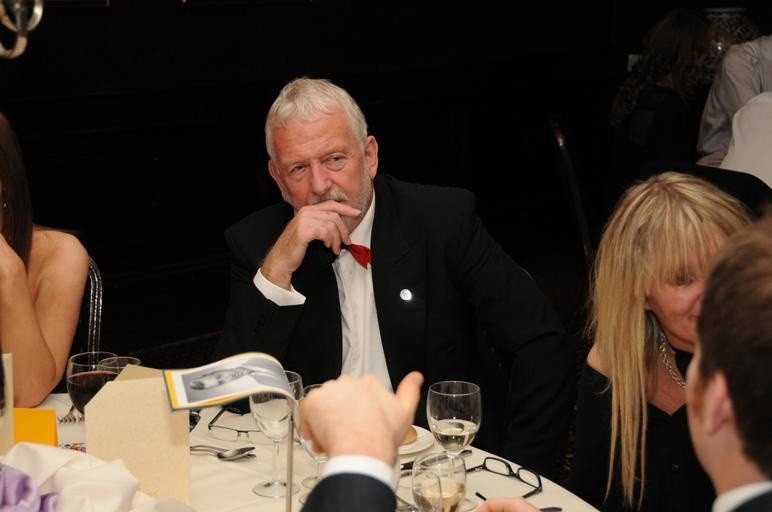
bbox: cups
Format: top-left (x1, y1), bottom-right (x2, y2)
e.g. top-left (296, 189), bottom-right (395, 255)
top-left (66, 351), bottom-right (142, 415)
top-left (411, 452), bottom-right (467, 512)
top-left (388, 470), bottom-right (442, 512)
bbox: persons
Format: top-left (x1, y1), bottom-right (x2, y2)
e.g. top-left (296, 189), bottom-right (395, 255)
top-left (0, 113), bottom-right (90, 409)
top-left (550, 168), bottom-right (755, 512)
top-left (290, 212), bottom-right (771, 510)
top-left (609, 8), bottom-right (772, 191)
top-left (187, 354), bottom-right (292, 392)
top-left (214, 75), bottom-right (580, 481)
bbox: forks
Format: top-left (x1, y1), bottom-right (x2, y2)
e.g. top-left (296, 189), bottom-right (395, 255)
top-left (57, 404), bottom-right (77, 424)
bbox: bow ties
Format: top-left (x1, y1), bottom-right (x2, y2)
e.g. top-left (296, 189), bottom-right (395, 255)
top-left (327, 242), bottom-right (371, 269)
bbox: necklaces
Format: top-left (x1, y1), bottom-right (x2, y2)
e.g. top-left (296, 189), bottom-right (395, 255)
top-left (657, 332), bottom-right (687, 392)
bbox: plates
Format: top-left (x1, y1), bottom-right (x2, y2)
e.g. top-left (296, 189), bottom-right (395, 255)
top-left (398, 425), bottom-right (434, 454)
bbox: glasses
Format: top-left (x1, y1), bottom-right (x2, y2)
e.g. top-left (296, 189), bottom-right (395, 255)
top-left (207, 404), bottom-right (290, 447)
top-left (427, 458), bottom-right (543, 503)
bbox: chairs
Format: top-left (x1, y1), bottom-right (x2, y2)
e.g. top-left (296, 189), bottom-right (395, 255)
top-left (68, 258), bottom-right (107, 365)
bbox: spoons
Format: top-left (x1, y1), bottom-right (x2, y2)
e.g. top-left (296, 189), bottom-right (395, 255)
top-left (190, 446), bottom-right (256, 461)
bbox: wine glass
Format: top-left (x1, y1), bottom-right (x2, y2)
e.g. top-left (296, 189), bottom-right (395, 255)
top-left (249, 370), bottom-right (304, 498)
top-left (426, 381), bottom-right (482, 512)
top-left (299, 384), bottom-right (330, 490)
